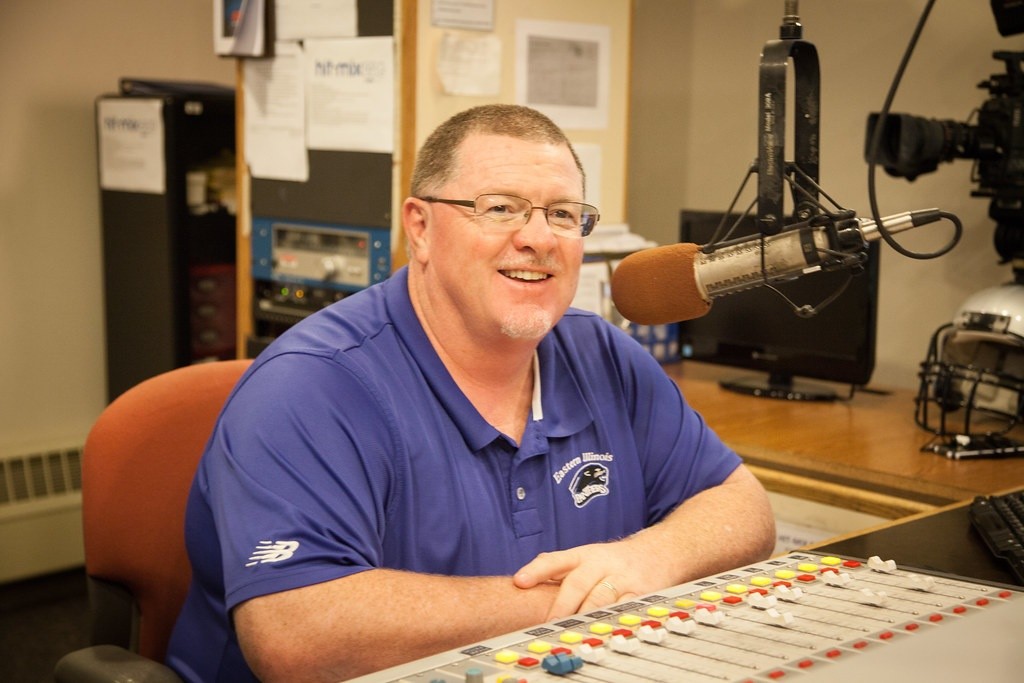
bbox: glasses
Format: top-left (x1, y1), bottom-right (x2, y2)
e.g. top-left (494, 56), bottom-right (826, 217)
top-left (417, 192), bottom-right (601, 239)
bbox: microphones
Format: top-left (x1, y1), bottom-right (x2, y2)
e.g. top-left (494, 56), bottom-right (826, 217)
top-left (610, 206), bottom-right (941, 326)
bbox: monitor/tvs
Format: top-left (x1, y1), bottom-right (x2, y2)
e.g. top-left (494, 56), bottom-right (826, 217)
top-left (677, 206), bottom-right (880, 401)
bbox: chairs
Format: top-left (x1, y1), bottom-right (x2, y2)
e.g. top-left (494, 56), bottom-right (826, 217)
top-left (80, 359), bottom-right (254, 667)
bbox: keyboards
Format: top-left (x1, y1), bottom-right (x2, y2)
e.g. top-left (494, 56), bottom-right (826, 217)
top-left (968, 488), bottom-right (1024, 588)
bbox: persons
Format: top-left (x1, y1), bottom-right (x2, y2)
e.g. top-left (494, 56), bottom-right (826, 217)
top-left (169, 105), bottom-right (778, 683)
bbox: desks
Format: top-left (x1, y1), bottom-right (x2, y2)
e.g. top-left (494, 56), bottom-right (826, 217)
top-left (648, 352), bottom-right (1024, 559)
